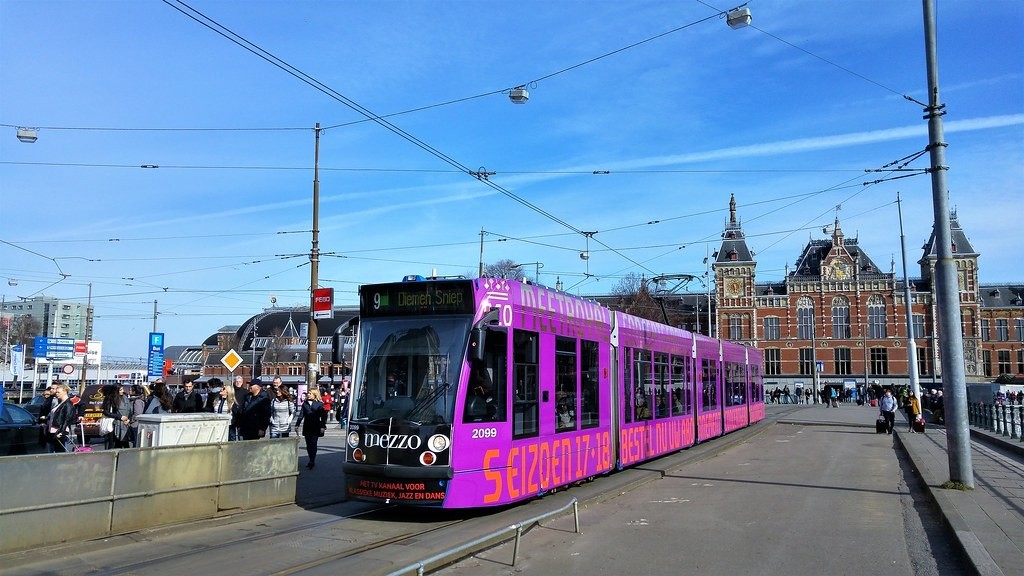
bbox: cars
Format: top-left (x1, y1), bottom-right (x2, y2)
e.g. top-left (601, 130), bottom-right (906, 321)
top-left (0, 401), bottom-right (49, 457)
top-left (23, 394), bottom-right (48, 418)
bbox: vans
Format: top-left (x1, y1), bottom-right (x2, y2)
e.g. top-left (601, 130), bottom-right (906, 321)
top-left (73, 384), bottom-right (152, 445)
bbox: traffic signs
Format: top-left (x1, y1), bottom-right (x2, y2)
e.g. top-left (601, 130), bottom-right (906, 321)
top-left (313, 288), bottom-right (335, 320)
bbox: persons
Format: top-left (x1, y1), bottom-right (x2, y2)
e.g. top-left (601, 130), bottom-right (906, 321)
top-left (23, 375), bottom-right (355, 471)
top-left (765, 381), bottom-right (945, 423)
top-left (879, 387), bottom-right (898, 435)
top-left (904, 389), bottom-right (921, 433)
top-left (993, 389), bottom-right (1024, 405)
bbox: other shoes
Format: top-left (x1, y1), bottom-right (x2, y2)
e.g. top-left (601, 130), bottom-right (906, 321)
top-left (306, 462), bottom-right (314, 469)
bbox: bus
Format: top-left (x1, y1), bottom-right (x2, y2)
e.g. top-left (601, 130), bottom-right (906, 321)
top-left (332, 268), bottom-right (765, 507)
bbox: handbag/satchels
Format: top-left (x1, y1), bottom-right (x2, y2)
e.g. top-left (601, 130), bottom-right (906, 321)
top-left (99, 406), bottom-right (113, 436)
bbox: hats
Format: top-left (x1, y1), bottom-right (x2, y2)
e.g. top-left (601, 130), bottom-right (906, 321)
top-left (246, 378), bottom-right (263, 387)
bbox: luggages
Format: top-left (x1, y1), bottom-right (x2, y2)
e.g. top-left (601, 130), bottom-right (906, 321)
top-left (871, 396), bottom-right (876, 406)
top-left (833, 399), bottom-right (838, 407)
top-left (913, 413), bottom-right (925, 433)
top-left (876, 415), bottom-right (886, 434)
top-left (55, 434), bottom-right (93, 453)
